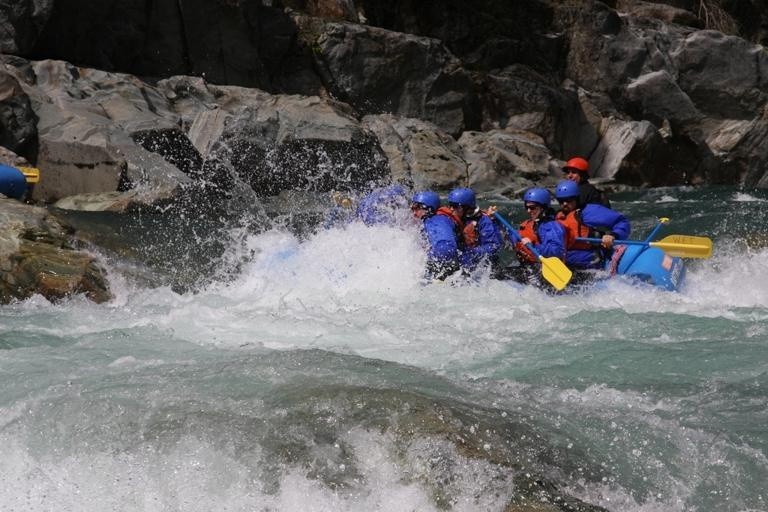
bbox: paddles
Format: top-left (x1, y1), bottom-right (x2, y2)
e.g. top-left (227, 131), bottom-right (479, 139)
top-left (16, 167), bottom-right (40, 184)
top-left (491, 213), bottom-right (572, 291)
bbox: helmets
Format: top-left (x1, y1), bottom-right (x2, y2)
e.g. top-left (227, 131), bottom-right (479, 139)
top-left (448, 187), bottom-right (475, 209)
top-left (524, 186), bottom-right (551, 206)
top-left (555, 179), bottom-right (580, 199)
top-left (412, 191), bottom-right (440, 211)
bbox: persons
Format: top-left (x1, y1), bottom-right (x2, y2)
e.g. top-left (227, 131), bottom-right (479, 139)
top-left (562, 156), bottom-right (611, 212)
top-left (325, 181), bottom-right (505, 285)
top-left (552, 179), bottom-right (631, 277)
top-left (485, 187), bottom-right (566, 298)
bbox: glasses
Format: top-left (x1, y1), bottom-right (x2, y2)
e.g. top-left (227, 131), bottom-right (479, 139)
top-left (450, 203), bottom-right (463, 208)
top-left (563, 157), bottom-right (589, 172)
top-left (525, 204), bottom-right (540, 211)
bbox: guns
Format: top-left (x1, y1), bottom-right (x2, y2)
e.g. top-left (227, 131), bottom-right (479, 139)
top-left (576, 235), bottom-right (713, 260)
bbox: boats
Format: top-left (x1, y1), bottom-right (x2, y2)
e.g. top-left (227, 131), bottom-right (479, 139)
top-left (0, 165), bottom-right (27, 199)
top-left (255, 238), bottom-right (683, 296)
